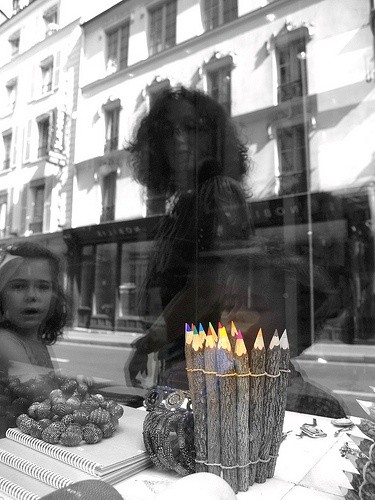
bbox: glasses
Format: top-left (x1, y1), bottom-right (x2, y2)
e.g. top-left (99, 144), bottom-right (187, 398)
top-left (158, 119), bottom-right (205, 139)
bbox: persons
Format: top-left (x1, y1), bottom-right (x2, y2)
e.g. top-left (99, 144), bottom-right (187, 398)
top-left (0, 242), bottom-right (75, 373)
top-left (126, 88), bottom-right (251, 396)
top-left (203, 239), bottom-right (343, 420)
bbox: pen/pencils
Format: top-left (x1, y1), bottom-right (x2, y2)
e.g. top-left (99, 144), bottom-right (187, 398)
top-left (180, 320), bottom-right (293, 493)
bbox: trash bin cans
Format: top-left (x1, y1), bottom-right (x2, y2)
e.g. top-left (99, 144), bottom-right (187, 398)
top-left (119, 284), bottom-right (136, 318)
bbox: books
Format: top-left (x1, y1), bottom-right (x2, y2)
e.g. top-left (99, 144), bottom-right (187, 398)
top-left (0, 403), bottom-right (155, 500)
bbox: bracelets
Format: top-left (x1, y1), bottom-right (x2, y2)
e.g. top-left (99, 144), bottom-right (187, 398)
top-left (142, 387), bottom-right (197, 477)
